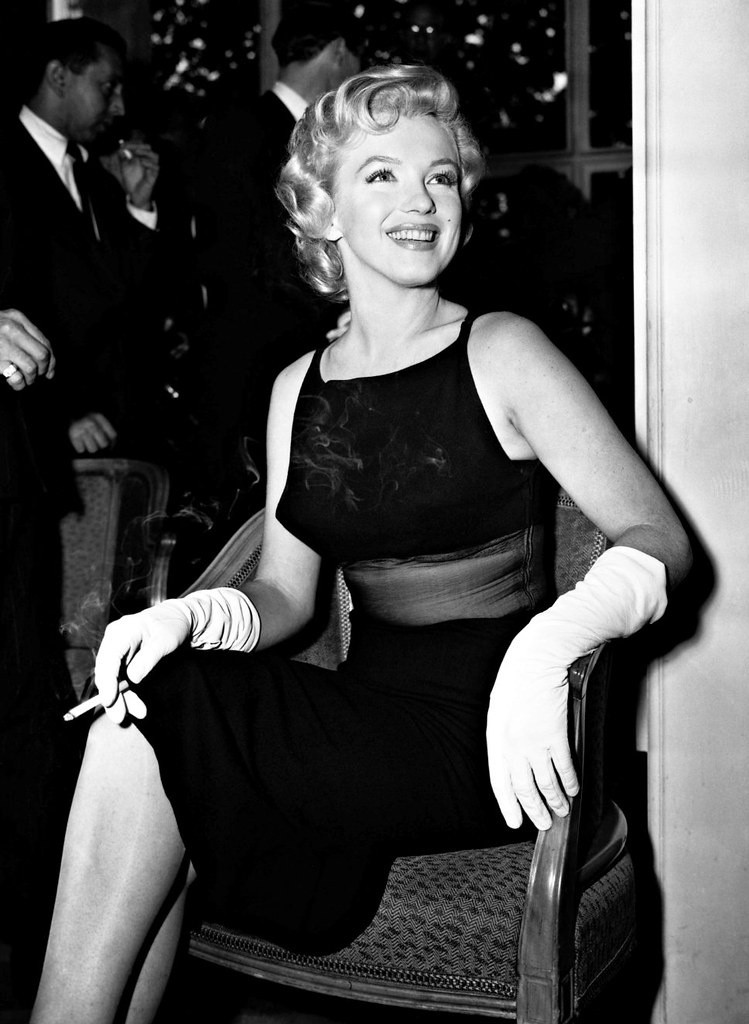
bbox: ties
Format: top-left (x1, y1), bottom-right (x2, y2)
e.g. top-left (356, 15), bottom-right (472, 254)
top-left (66, 140), bottom-right (97, 240)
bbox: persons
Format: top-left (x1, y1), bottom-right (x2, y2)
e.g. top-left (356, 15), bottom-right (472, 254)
top-left (32, 64), bottom-right (694, 1024)
top-left (0, 0), bottom-right (375, 575)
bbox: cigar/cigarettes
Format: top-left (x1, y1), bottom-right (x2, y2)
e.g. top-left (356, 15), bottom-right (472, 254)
top-left (118, 138), bottom-right (132, 161)
top-left (63, 680), bottom-right (128, 721)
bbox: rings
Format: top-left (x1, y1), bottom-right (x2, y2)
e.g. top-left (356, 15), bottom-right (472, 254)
top-left (5, 368), bottom-right (16, 379)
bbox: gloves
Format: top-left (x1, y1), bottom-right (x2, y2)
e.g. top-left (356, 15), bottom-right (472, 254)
top-left (90, 581), bottom-right (264, 729)
top-left (483, 544), bottom-right (670, 835)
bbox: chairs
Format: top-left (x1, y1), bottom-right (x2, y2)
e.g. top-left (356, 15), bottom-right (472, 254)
top-left (173, 489), bottom-right (638, 1024)
